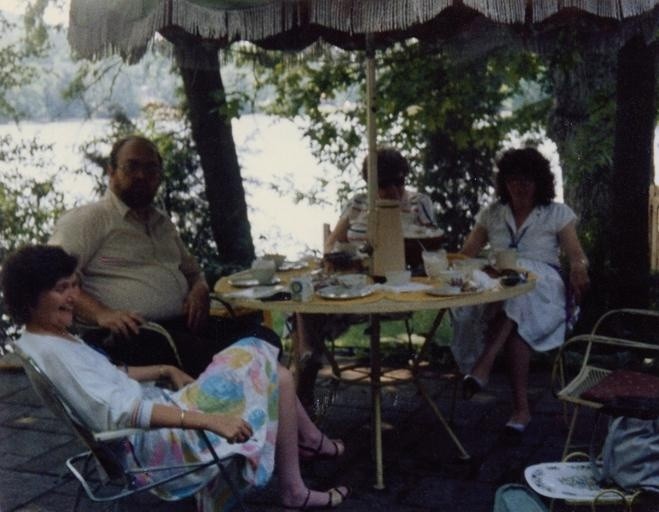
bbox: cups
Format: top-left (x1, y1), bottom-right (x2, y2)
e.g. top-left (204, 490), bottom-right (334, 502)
top-left (407, 232), bottom-right (442, 276)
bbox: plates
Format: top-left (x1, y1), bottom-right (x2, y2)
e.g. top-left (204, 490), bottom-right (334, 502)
top-left (225, 249), bottom-right (520, 299)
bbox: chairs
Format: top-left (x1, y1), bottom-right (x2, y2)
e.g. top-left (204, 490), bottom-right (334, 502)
top-left (3, 340), bottom-right (269, 512)
top-left (298, 223), bottom-right (429, 377)
top-left (551, 308), bottom-right (659, 466)
top-left (450, 237), bottom-right (594, 427)
top-left (72, 290), bottom-right (248, 394)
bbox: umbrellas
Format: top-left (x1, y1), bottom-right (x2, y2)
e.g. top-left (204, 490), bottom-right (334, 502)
top-left (69, 0), bottom-right (659, 491)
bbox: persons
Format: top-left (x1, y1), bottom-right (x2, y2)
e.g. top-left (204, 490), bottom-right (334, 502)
top-left (292, 149), bottom-right (435, 408)
top-left (49, 135), bottom-right (284, 378)
top-left (454, 147), bottom-right (591, 433)
top-left (0, 244), bottom-right (352, 509)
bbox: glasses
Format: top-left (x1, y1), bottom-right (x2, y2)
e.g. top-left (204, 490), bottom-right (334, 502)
top-left (115, 163), bottom-right (164, 177)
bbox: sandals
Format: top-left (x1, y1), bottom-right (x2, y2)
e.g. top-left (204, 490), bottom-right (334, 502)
top-left (504, 414), bottom-right (532, 433)
top-left (296, 431), bottom-right (351, 465)
top-left (462, 373), bottom-right (490, 393)
top-left (281, 483), bottom-right (353, 512)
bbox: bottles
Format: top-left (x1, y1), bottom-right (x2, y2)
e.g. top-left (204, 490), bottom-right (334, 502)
top-left (372, 199), bottom-right (406, 285)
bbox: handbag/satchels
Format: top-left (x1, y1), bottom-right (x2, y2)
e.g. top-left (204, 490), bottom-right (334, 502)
top-left (595, 416), bottom-right (659, 494)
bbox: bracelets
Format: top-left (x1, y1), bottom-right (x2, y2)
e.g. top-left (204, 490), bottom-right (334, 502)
top-left (159, 364), bottom-right (164, 378)
top-left (180, 409), bottom-right (185, 430)
